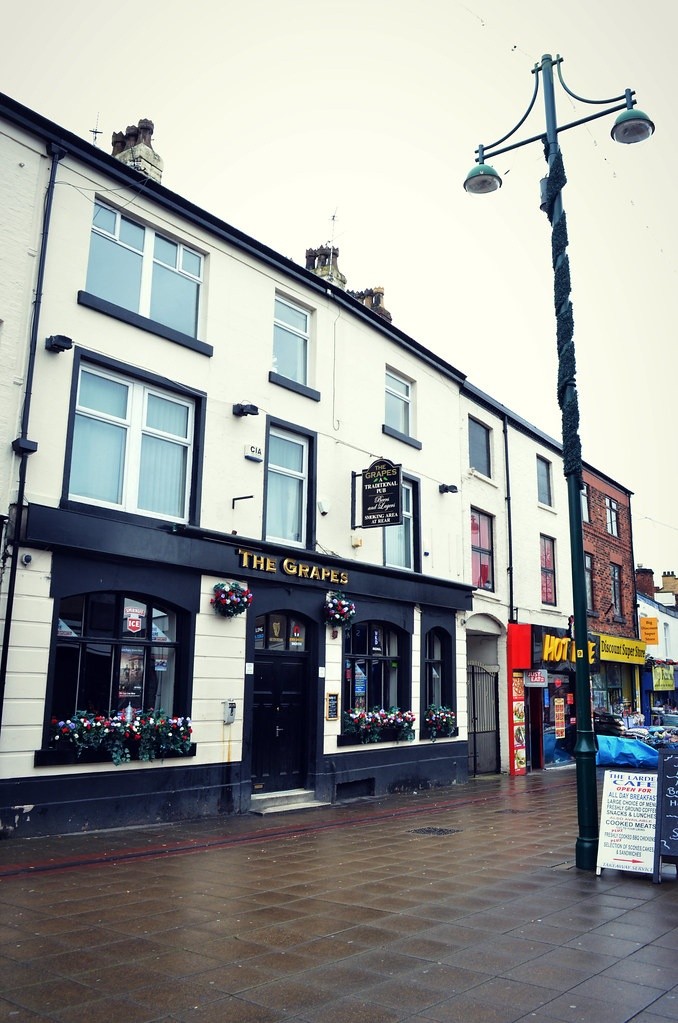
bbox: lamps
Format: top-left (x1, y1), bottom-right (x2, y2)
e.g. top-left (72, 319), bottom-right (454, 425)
top-left (233, 403), bottom-right (259, 417)
top-left (44, 334), bottom-right (72, 354)
top-left (439, 484), bottom-right (459, 494)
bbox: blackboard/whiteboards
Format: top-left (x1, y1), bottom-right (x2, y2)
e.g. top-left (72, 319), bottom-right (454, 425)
top-left (654, 748), bottom-right (678, 856)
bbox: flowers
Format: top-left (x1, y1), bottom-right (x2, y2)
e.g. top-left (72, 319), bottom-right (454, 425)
top-left (342, 702), bottom-right (416, 745)
top-left (209, 581), bottom-right (254, 621)
top-left (50, 705), bottom-right (193, 767)
top-left (424, 703), bottom-right (456, 742)
top-left (325, 591), bottom-right (355, 630)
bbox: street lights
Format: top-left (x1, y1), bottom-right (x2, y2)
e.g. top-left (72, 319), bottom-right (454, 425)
top-left (458, 52), bottom-right (659, 872)
